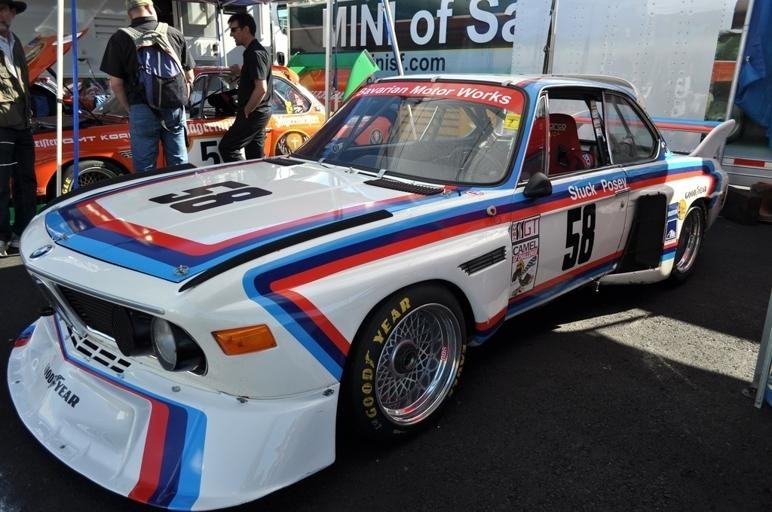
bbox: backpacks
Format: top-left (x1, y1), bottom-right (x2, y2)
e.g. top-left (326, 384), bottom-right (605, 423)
top-left (118, 21), bottom-right (192, 113)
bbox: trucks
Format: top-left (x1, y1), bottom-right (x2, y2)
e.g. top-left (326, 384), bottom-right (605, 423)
top-left (262, 2), bottom-right (772, 222)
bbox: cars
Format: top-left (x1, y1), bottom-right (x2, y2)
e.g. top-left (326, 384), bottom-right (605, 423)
top-left (6, 29), bottom-right (339, 204)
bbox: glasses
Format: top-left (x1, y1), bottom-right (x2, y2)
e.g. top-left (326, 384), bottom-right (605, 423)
top-left (231, 26), bottom-right (240, 32)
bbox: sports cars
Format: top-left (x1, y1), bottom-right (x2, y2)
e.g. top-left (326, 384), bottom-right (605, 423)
top-left (6, 71), bottom-right (739, 511)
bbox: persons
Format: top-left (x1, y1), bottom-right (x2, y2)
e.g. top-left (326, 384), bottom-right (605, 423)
top-left (1, 2), bottom-right (36, 258)
top-left (99, 1), bottom-right (197, 175)
top-left (217, 12), bottom-right (273, 164)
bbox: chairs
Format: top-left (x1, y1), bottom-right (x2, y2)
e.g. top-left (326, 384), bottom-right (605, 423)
top-left (523, 112), bottom-right (581, 180)
